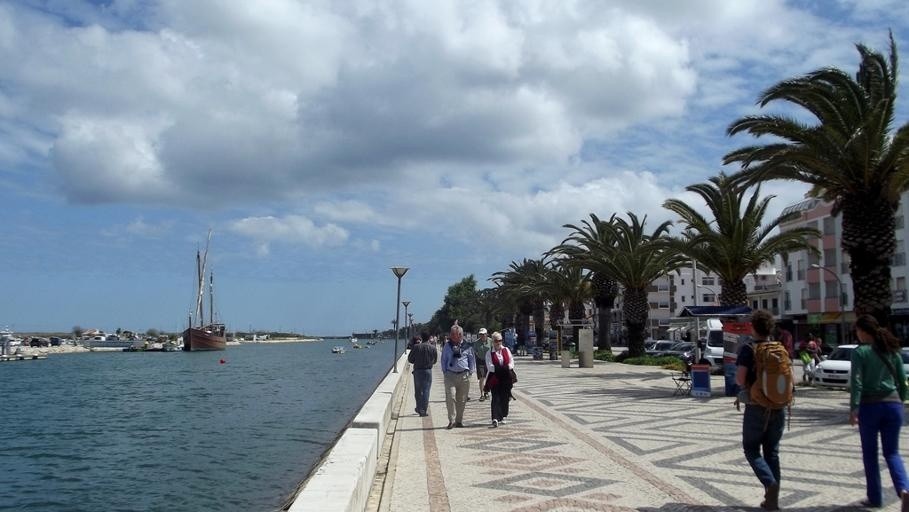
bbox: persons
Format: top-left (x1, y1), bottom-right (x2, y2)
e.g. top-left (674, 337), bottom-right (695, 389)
top-left (799, 333), bottom-right (824, 384)
top-left (735, 309), bottom-right (793, 510)
top-left (405, 323), bottom-right (519, 429)
top-left (848, 314), bottom-right (909, 512)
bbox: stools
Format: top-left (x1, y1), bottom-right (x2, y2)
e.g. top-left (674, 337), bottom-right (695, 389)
top-left (672, 378), bottom-right (692, 396)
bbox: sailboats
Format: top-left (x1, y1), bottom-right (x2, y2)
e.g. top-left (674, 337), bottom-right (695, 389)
top-left (182, 225), bottom-right (226, 351)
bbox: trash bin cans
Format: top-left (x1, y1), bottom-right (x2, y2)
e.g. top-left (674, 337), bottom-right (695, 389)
top-left (532, 346), bottom-right (543, 360)
top-left (560, 351), bottom-right (570, 368)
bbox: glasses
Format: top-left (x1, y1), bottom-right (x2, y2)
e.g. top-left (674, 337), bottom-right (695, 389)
top-left (493, 339), bottom-right (502, 343)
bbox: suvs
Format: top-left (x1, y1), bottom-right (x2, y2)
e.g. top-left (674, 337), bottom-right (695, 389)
top-left (29, 336), bottom-right (62, 347)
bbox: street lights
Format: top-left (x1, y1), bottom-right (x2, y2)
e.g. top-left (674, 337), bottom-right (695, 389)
top-left (389, 266), bottom-right (411, 361)
top-left (401, 301), bottom-right (417, 347)
top-left (811, 263), bottom-right (846, 344)
top-left (697, 285), bottom-right (720, 306)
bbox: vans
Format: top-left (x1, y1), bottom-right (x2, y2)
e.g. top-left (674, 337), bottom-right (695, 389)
top-left (703, 318), bottom-right (725, 374)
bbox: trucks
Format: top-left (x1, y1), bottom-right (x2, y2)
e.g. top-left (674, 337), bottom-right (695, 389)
top-left (146, 335), bottom-right (168, 343)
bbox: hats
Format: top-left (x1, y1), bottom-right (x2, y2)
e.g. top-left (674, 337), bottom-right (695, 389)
top-left (478, 328), bottom-right (487, 334)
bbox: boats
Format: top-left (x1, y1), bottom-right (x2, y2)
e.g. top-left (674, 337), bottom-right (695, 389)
top-left (333, 337), bottom-right (378, 352)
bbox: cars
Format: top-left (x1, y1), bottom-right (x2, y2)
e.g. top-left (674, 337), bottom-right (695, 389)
top-left (129, 334), bottom-right (147, 341)
top-left (645, 341), bottom-right (706, 365)
top-left (898, 347), bottom-right (909, 373)
top-left (812, 344), bottom-right (860, 391)
top-left (89, 334), bottom-right (120, 341)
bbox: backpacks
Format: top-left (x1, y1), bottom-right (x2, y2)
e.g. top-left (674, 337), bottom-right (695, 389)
top-left (745, 339), bottom-right (794, 410)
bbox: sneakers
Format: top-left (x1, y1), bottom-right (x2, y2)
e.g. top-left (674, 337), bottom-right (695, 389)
top-left (492, 418), bottom-right (498, 427)
top-left (415, 407), bottom-right (428, 416)
top-left (859, 498), bottom-right (880, 507)
top-left (501, 416), bottom-right (507, 425)
top-left (761, 482), bottom-right (779, 511)
top-left (447, 421), bottom-right (464, 429)
top-left (900, 490), bottom-right (909, 511)
top-left (479, 392), bottom-right (490, 402)
